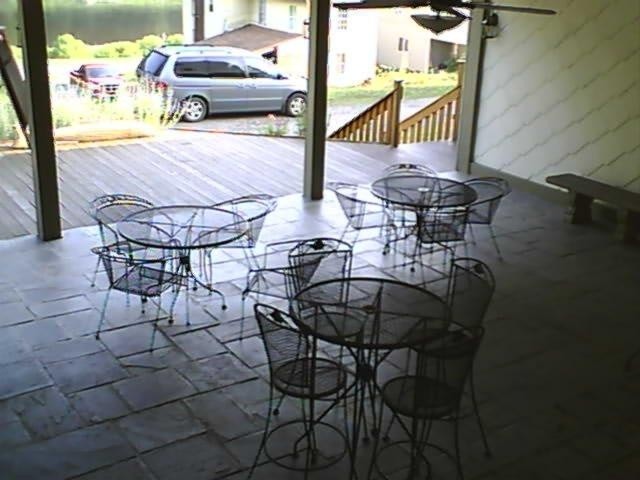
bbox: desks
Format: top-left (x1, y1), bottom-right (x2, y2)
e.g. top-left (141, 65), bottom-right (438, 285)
top-left (118, 205), bottom-right (249, 324)
top-left (370, 175), bottom-right (479, 271)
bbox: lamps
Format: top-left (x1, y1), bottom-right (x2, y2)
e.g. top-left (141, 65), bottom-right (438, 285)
top-left (479, 13), bottom-right (500, 42)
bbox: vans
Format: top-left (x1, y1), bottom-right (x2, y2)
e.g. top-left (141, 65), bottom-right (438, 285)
top-left (135, 43), bottom-right (310, 123)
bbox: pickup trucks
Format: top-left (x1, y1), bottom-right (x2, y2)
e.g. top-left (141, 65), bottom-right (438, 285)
top-left (70, 64), bottom-right (126, 100)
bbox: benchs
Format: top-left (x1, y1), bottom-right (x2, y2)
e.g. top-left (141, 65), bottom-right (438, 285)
top-left (542, 170), bottom-right (640, 248)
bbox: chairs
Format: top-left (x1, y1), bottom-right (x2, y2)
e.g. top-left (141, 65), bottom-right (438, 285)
top-left (327, 182), bottom-right (397, 254)
top-left (240, 239), bottom-right (494, 479)
top-left (443, 176), bottom-right (512, 265)
top-left (89, 194), bottom-right (188, 353)
top-left (199, 194), bottom-right (279, 294)
top-left (379, 163), bottom-right (442, 244)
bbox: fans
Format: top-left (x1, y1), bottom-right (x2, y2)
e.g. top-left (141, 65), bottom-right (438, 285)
top-left (328, 1), bottom-right (559, 37)
